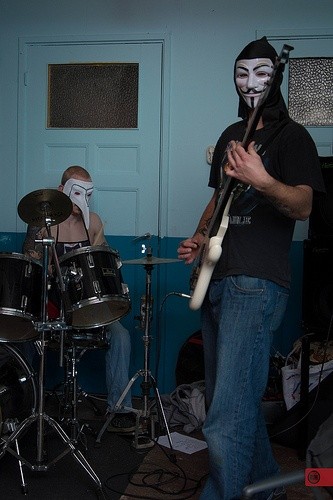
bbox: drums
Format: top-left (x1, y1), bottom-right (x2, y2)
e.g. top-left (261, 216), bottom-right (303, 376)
top-left (51, 316), bottom-right (113, 351)
top-left (0, 341), bottom-right (40, 459)
top-left (52, 244), bottom-right (133, 329)
top-left (0, 251), bottom-right (50, 343)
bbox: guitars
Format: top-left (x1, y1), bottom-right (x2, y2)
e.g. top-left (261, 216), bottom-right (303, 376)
top-left (187, 42), bottom-right (295, 311)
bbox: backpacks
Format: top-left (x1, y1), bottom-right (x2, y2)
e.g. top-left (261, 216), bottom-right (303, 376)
top-left (305, 413), bottom-right (333, 500)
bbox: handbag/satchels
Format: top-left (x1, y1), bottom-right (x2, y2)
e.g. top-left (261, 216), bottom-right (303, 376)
top-left (281, 341), bottom-right (333, 410)
top-left (178, 382), bottom-right (207, 433)
top-left (157, 397), bottom-right (188, 435)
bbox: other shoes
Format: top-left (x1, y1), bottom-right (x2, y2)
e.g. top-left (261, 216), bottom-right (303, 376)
top-left (106, 412), bottom-right (136, 428)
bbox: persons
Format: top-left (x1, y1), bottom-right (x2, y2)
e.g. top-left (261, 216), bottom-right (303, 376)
top-left (177, 35), bottom-right (319, 500)
top-left (22, 166), bottom-right (150, 433)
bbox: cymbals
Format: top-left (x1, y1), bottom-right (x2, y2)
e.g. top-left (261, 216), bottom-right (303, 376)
top-left (17, 188), bottom-right (73, 228)
top-left (120, 257), bottom-right (184, 265)
